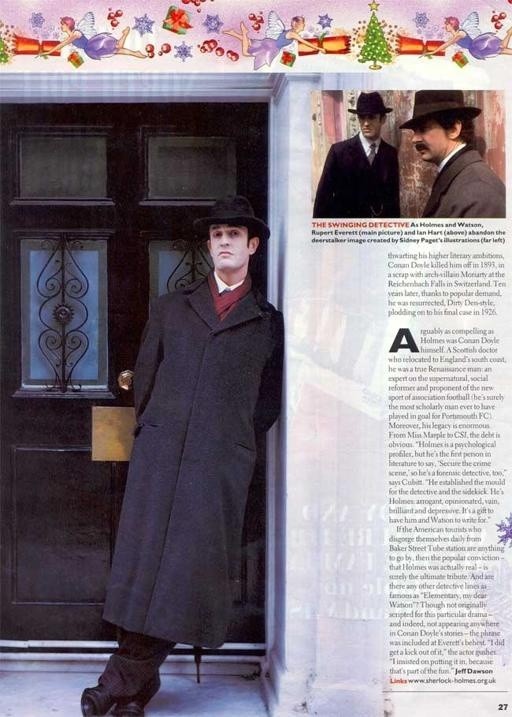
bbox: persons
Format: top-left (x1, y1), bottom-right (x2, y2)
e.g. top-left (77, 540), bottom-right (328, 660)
top-left (80, 189), bottom-right (288, 715)
top-left (396, 91), bottom-right (504, 220)
top-left (313, 90), bottom-right (406, 216)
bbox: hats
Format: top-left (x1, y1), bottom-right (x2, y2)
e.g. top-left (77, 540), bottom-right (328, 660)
top-left (398, 90), bottom-right (481, 131)
top-left (343, 91), bottom-right (393, 116)
top-left (191, 195), bottom-right (270, 244)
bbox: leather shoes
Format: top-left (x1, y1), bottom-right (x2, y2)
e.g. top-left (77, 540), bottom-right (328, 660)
top-left (110, 702), bottom-right (146, 717)
top-left (80, 682), bottom-right (110, 717)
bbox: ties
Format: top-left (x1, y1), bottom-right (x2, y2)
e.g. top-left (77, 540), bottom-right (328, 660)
top-left (367, 143), bottom-right (377, 164)
